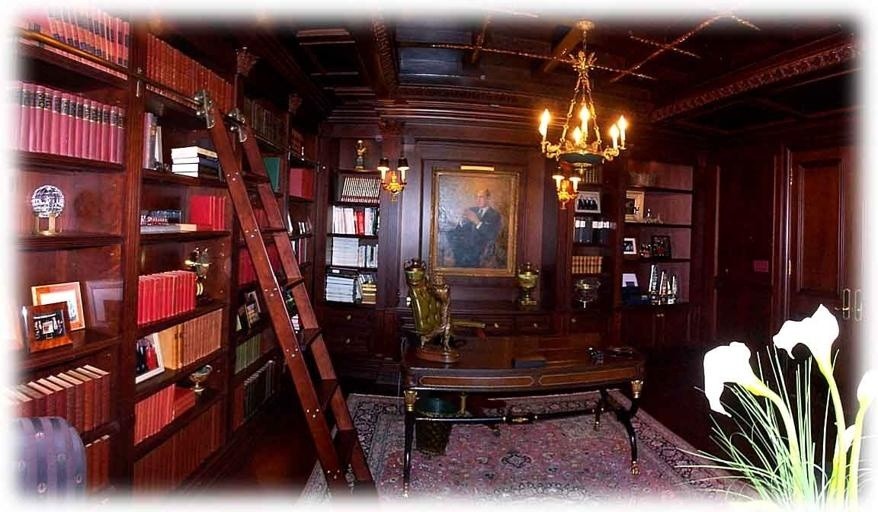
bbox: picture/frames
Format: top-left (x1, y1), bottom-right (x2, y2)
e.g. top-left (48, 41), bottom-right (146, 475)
top-left (625, 191), bottom-right (645, 223)
top-left (623, 232), bottom-right (672, 260)
top-left (428, 164), bottom-right (520, 280)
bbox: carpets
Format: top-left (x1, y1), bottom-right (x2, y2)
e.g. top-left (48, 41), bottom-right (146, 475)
top-left (297, 388), bottom-right (762, 504)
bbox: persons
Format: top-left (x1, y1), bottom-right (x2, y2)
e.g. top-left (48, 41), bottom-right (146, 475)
top-left (419, 273), bottom-right (454, 354)
top-left (233, 291), bottom-right (304, 427)
top-left (136, 339), bottom-right (159, 374)
top-left (623, 234), bottom-right (669, 259)
top-left (444, 188), bottom-right (503, 267)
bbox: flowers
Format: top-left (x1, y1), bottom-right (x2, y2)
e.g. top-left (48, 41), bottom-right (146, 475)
top-left (667, 301), bottom-right (874, 506)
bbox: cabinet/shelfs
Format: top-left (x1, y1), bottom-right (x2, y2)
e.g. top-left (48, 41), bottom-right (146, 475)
top-left (20, 7), bottom-right (400, 498)
top-left (553, 155), bottom-right (722, 361)
top-left (381, 299), bottom-right (556, 370)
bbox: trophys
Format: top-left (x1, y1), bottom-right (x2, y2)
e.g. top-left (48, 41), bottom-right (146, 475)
top-left (515, 261), bottom-right (540, 307)
top-left (354, 140), bottom-right (367, 169)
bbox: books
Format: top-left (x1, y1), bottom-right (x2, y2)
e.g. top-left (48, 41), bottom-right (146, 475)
top-left (244, 98), bottom-right (310, 157)
top-left (14, 6), bottom-right (130, 81)
top-left (146, 31), bottom-right (234, 118)
top-left (160, 307), bottom-right (222, 370)
top-left (241, 193), bottom-right (283, 241)
top-left (572, 166), bottom-right (611, 274)
top-left (289, 168), bottom-right (316, 201)
top-left (134, 381), bottom-right (197, 444)
top-left (138, 270), bottom-right (197, 324)
top-left (263, 157), bottom-right (282, 191)
top-left (15, 364), bottom-right (112, 494)
top-left (133, 400), bottom-right (226, 496)
top-left (289, 205), bottom-right (314, 265)
top-left (140, 210), bottom-right (197, 234)
top-left (323, 176), bottom-right (383, 304)
top-left (239, 243), bottom-right (281, 286)
top-left (143, 112), bottom-right (226, 182)
top-left (14, 81), bottom-right (126, 163)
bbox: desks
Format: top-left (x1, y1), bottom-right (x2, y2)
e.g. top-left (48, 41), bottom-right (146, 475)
top-left (397, 332), bottom-right (645, 499)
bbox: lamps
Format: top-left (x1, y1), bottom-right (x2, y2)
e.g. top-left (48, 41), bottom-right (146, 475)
top-left (536, 21), bottom-right (632, 212)
top-left (376, 155), bottom-right (411, 203)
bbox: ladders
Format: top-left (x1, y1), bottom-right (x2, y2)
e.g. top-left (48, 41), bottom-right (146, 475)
top-left (192, 87), bottom-right (384, 496)
